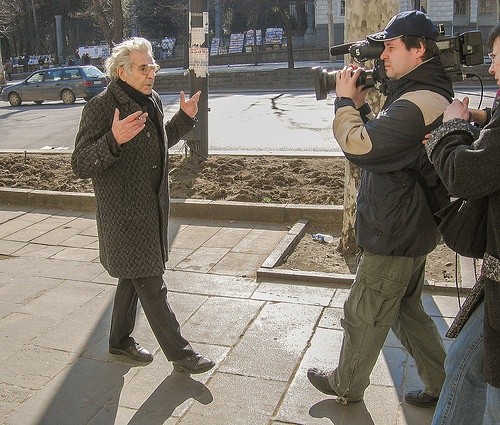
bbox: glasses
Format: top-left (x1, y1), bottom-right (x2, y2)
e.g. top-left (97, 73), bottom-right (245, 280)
top-left (131, 65), bottom-right (160, 74)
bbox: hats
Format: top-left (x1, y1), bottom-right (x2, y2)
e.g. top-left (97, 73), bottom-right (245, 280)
top-left (366, 10), bottom-right (437, 41)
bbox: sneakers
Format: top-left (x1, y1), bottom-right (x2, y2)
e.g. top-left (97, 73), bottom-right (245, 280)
top-left (307, 367), bottom-right (338, 395)
top-left (405, 390), bottom-right (438, 407)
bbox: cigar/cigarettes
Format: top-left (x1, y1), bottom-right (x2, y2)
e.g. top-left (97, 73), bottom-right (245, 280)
top-left (136, 117), bottom-right (143, 120)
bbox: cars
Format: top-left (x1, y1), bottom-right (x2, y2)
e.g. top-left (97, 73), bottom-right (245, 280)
top-left (1, 64), bottom-right (107, 107)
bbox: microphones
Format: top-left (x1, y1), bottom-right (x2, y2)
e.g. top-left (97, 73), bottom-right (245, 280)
top-left (330, 42), bottom-right (355, 56)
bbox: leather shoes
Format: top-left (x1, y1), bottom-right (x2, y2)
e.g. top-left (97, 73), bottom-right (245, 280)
top-left (173, 352), bottom-right (215, 374)
top-left (109, 338), bottom-right (153, 362)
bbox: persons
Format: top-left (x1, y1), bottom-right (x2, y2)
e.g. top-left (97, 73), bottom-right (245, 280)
top-left (38, 56), bottom-right (44, 70)
top-left (426, 24), bottom-right (500, 425)
top-left (18, 57), bottom-right (23, 65)
top-left (70, 36), bottom-right (216, 374)
top-left (22, 55), bottom-right (29, 72)
top-left (307, 10), bottom-right (455, 408)
top-left (4, 59), bottom-right (13, 81)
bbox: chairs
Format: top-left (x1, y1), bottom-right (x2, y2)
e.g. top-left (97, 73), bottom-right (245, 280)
top-left (58, 73), bottom-right (67, 80)
top-left (42, 74), bottom-right (54, 82)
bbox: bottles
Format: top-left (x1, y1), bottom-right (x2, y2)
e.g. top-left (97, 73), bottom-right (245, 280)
top-left (312, 233), bottom-right (334, 243)
